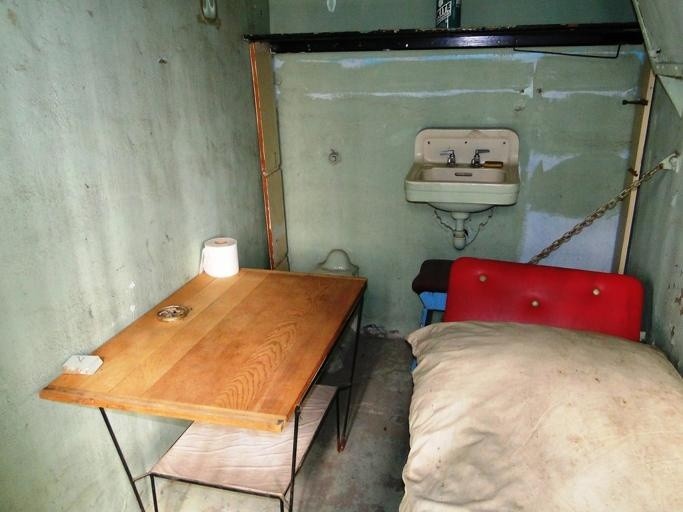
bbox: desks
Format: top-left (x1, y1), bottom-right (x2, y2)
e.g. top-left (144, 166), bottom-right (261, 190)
top-left (39, 267), bottom-right (367, 511)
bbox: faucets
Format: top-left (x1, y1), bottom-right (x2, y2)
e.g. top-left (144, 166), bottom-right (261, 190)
top-left (440, 150), bottom-right (455, 166)
top-left (471, 150), bottom-right (490, 167)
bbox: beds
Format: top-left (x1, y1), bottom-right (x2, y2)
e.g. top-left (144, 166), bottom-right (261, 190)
top-left (402, 257), bottom-right (682, 510)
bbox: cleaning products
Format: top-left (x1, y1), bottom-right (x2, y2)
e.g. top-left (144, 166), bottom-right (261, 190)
top-left (436, 0), bottom-right (461, 29)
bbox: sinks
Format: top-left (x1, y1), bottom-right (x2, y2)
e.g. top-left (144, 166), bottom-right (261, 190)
top-left (405, 163), bottom-right (520, 213)
